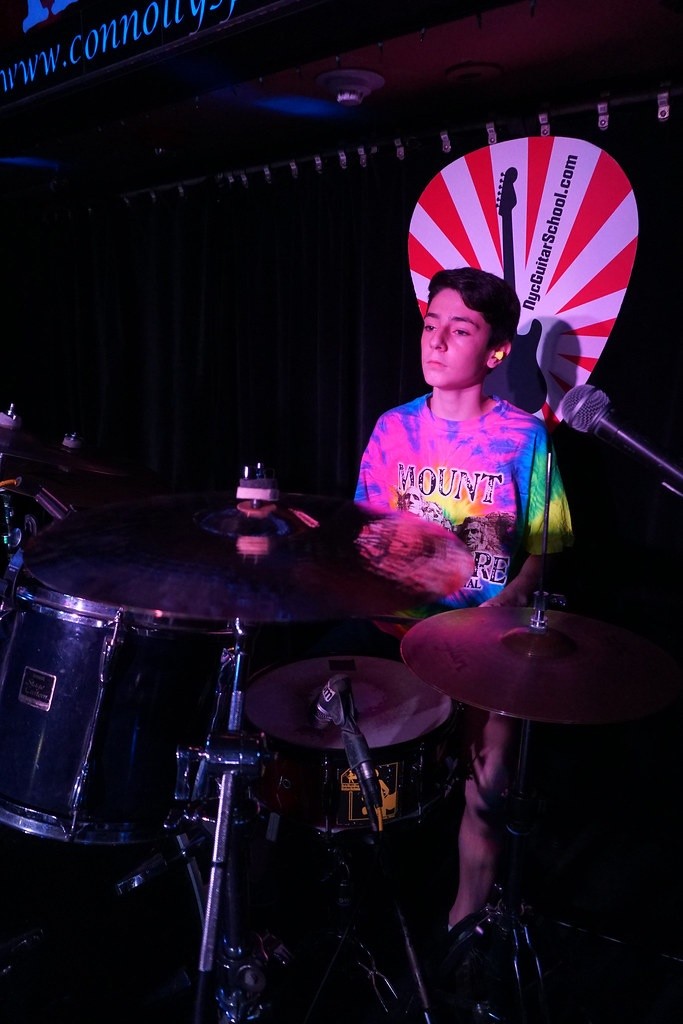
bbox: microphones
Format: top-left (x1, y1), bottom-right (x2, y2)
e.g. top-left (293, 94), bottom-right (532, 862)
top-left (561, 384), bottom-right (683, 488)
top-left (308, 674), bottom-right (351, 730)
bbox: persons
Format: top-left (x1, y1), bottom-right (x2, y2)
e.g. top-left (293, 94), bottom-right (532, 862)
top-left (355, 266), bottom-right (577, 973)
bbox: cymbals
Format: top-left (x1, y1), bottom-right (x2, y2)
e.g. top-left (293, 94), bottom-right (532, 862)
top-left (2, 454), bottom-right (169, 508)
top-left (0, 408), bottom-right (128, 476)
top-left (404, 604), bottom-right (675, 727)
top-left (27, 495), bottom-right (475, 622)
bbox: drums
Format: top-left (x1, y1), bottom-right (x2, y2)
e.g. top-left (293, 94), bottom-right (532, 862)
top-left (246, 656), bottom-right (461, 829)
top-left (0, 580), bottom-right (251, 844)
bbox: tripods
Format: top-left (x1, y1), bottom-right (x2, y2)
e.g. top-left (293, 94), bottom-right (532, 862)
top-left (0, 591), bottom-right (556, 1024)
